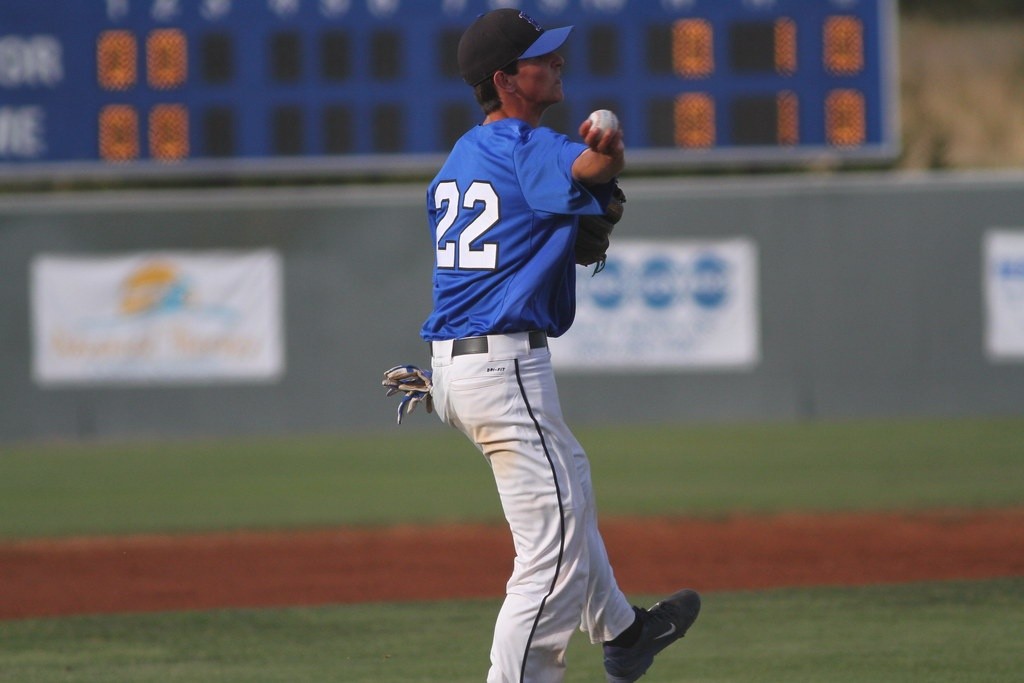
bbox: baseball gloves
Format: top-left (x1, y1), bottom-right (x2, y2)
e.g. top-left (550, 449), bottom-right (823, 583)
top-left (577, 184), bottom-right (625, 265)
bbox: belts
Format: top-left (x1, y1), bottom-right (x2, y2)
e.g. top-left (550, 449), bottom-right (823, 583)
top-left (430, 331), bottom-right (548, 357)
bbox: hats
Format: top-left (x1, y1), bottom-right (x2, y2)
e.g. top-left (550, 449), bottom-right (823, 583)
top-left (457, 8), bottom-right (574, 86)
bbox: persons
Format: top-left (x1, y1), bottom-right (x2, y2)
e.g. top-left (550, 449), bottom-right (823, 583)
top-left (420, 9), bottom-right (701, 683)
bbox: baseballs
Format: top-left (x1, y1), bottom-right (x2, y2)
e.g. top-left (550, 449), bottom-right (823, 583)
top-left (589, 109), bottom-right (621, 141)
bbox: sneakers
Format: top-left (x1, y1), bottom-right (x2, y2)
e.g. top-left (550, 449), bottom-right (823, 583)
top-left (603, 589), bottom-right (701, 683)
